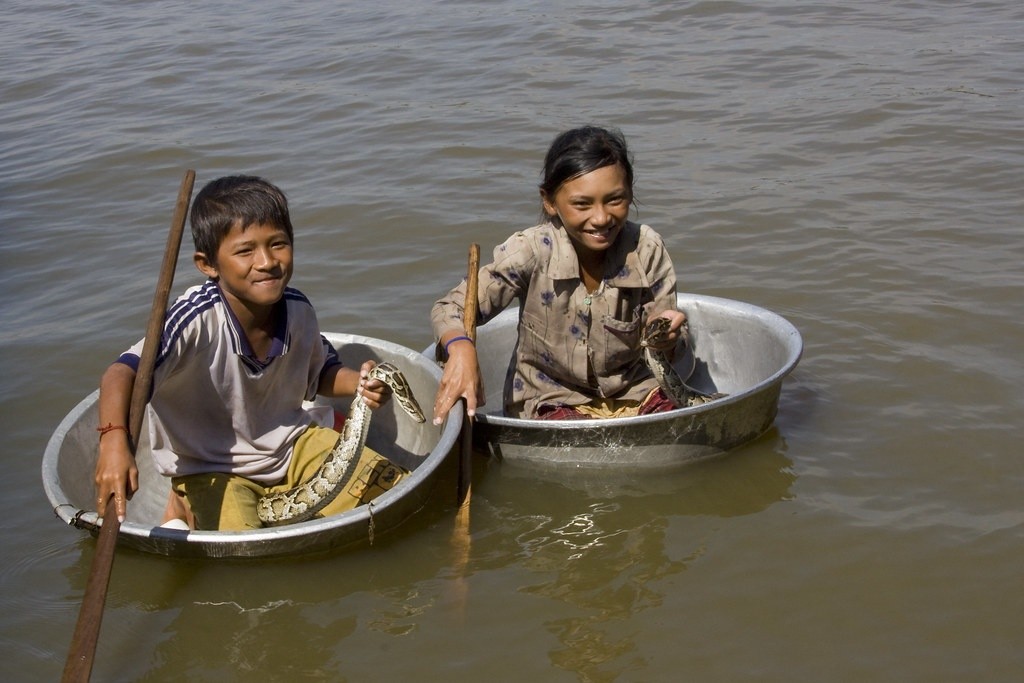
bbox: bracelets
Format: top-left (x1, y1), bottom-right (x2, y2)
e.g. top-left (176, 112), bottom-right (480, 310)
top-left (97, 423), bottom-right (130, 441)
top-left (445, 335), bottom-right (474, 353)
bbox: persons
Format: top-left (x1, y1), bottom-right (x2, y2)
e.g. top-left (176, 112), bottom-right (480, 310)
top-left (93, 174), bottom-right (414, 534)
top-left (432, 127), bottom-right (695, 427)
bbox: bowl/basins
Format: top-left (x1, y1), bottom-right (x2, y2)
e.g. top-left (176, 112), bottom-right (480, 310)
top-left (420, 292), bottom-right (804, 468)
top-left (41, 331), bottom-right (464, 569)
